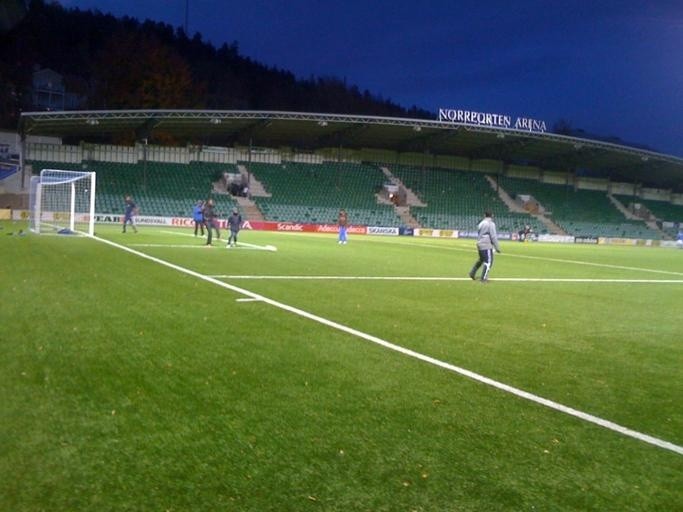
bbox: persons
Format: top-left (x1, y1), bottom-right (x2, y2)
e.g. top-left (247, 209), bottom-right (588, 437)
top-left (337, 208), bottom-right (349, 245)
top-left (120, 196), bottom-right (140, 234)
top-left (224, 207), bottom-right (243, 249)
top-left (468, 210), bottom-right (501, 284)
top-left (193, 200), bottom-right (206, 236)
top-left (203, 199), bottom-right (223, 248)
